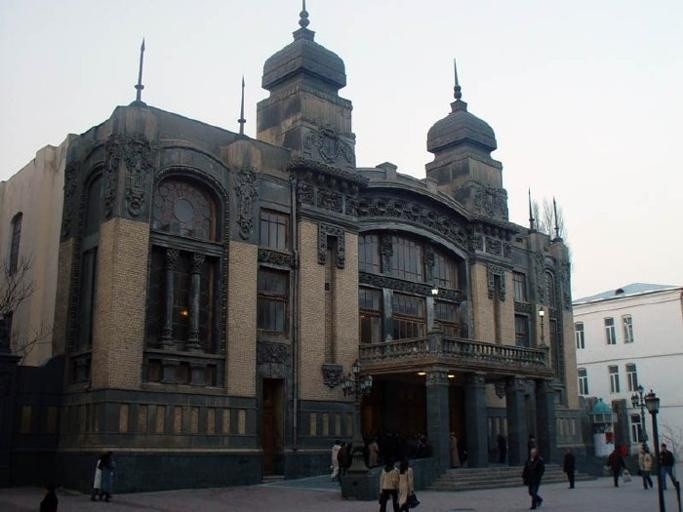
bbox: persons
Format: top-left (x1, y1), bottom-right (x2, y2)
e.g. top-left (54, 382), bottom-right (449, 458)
top-left (39, 481), bottom-right (57, 512)
top-left (563, 448), bottom-right (575, 489)
top-left (639, 440), bottom-right (654, 489)
top-left (330, 439), bottom-right (379, 481)
top-left (660, 443), bottom-right (678, 489)
top-left (521, 437), bottom-right (545, 509)
top-left (379, 457), bottom-right (414, 512)
top-left (449, 432), bottom-right (466, 466)
top-left (496, 434), bottom-right (506, 463)
top-left (608, 448), bottom-right (626, 487)
top-left (90, 451), bottom-right (118, 502)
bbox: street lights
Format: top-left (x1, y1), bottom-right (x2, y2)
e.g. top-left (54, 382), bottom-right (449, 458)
top-left (645, 389), bottom-right (666, 512)
top-left (342, 358), bottom-right (374, 473)
top-left (630, 384), bottom-right (648, 454)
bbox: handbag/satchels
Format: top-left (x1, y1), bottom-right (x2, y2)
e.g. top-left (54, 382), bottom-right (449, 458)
top-left (623, 472), bottom-right (631, 482)
top-left (407, 491), bottom-right (420, 508)
top-left (379, 493), bottom-right (391, 504)
